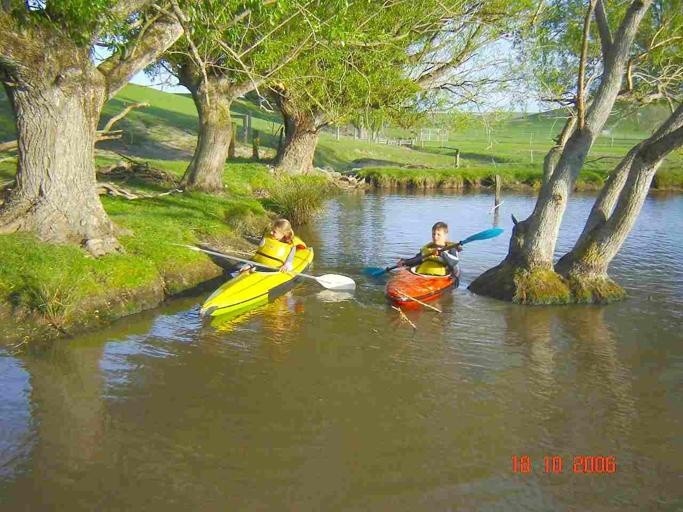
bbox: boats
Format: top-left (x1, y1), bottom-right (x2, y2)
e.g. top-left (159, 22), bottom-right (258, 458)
top-left (200, 246), bottom-right (314, 316)
top-left (384, 262), bottom-right (461, 311)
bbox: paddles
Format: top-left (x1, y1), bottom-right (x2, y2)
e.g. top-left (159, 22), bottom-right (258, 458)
top-left (371, 227), bottom-right (503, 276)
top-left (191, 247), bottom-right (356, 290)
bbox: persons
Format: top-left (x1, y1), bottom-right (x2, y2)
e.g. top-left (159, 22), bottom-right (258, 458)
top-left (239, 219), bottom-right (295, 272)
top-left (397, 222), bottom-right (459, 275)
